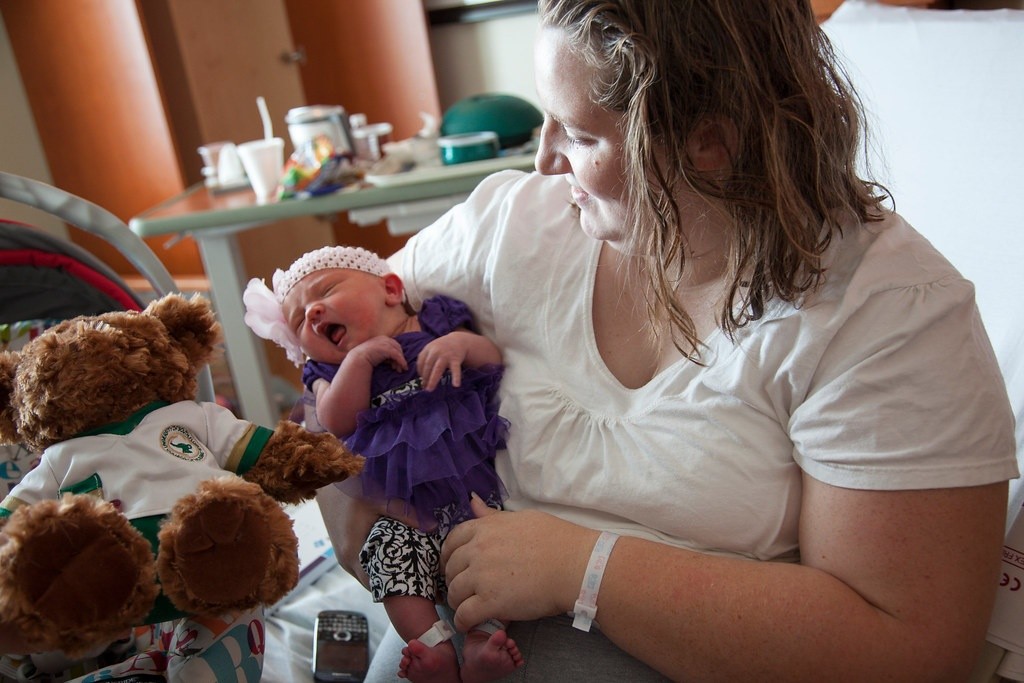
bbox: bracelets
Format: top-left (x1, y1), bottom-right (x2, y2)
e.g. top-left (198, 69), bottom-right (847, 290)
top-left (571, 530), bottom-right (621, 633)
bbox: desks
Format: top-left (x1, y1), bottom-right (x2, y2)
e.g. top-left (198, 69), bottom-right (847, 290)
top-left (130, 118), bottom-right (535, 427)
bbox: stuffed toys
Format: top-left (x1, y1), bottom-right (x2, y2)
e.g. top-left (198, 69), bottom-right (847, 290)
top-left (0, 291), bottom-right (365, 660)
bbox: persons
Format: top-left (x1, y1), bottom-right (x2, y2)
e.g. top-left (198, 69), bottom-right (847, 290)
top-left (244, 246), bottom-right (524, 683)
top-left (302, 0), bottom-right (1024, 683)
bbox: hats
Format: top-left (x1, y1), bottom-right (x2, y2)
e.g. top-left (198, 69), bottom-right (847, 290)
top-left (243, 245), bottom-right (407, 368)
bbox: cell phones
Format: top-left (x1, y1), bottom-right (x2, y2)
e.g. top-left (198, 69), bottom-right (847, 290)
top-left (311, 609), bottom-right (370, 683)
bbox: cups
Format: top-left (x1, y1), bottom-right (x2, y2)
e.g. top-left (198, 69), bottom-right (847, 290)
top-left (237, 137), bottom-right (287, 203)
top-left (435, 130), bottom-right (500, 165)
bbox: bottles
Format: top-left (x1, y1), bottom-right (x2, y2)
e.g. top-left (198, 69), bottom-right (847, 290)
top-left (197, 104), bottom-right (392, 192)
